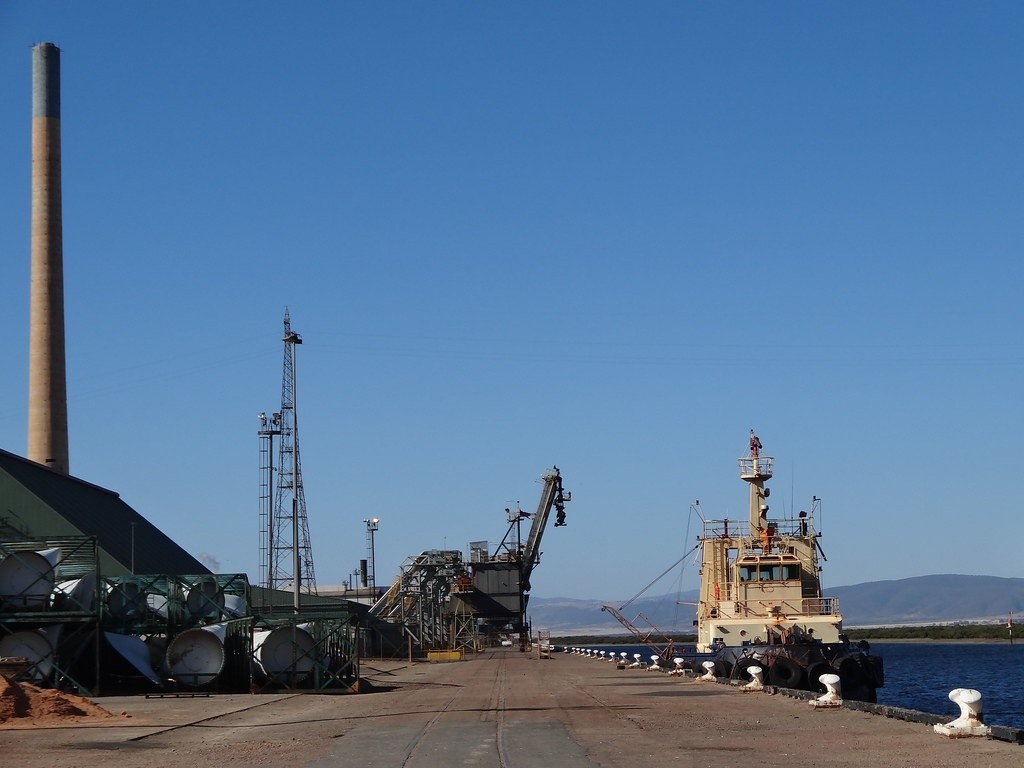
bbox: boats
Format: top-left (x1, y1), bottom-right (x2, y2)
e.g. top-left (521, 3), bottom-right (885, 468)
top-left (695, 429), bottom-right (877, 695)
